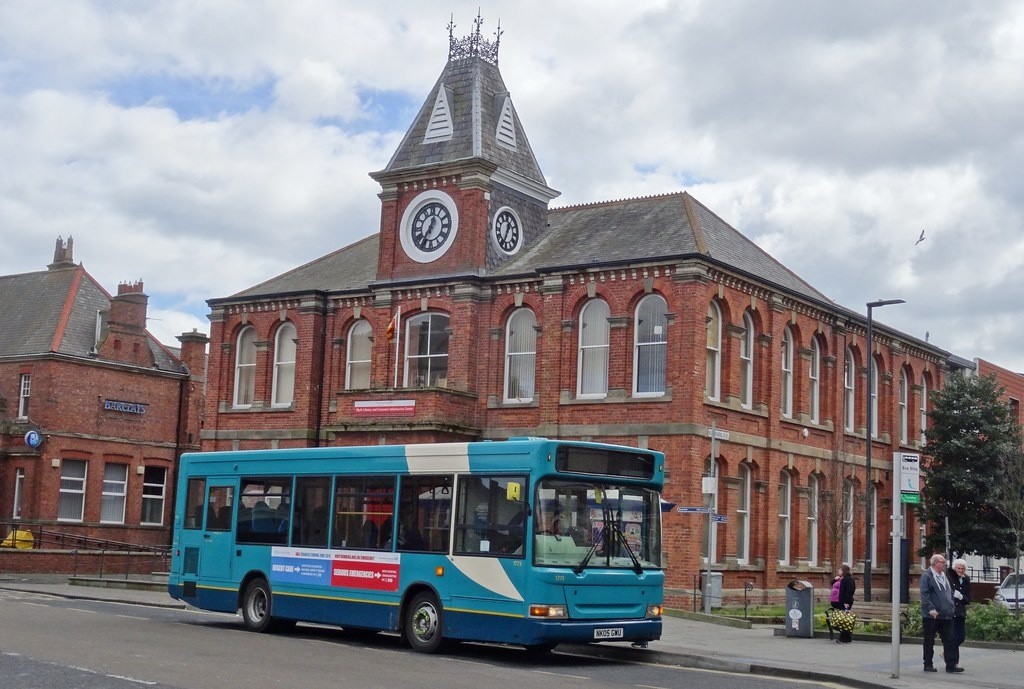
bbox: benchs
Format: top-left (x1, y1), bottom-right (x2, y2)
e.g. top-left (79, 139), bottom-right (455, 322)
top-left (825, 600), bottom-right (909, 644)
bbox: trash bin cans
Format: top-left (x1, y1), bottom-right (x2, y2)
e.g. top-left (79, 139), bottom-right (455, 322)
top-left (786, 581), bottom-right (814, 638)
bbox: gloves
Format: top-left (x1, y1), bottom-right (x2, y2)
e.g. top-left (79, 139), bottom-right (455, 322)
top-left (954, 590), bottom-right (964, 601)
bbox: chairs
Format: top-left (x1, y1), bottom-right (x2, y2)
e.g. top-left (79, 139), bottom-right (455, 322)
top-left (274, 502), bottom-right (290, 530)
top-left (227, 500), bottom-right (250, 532)
top-left (362, 520), bottom-right (379, 549)
top-left (251, 501), bottom-right (276, 533)
top-left (194, 505), bottom-right (218, 528)
top-left (218, 506), bottom-right (232, 529)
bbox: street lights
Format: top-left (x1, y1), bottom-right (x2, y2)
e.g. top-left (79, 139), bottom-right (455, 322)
top-left (862, 299), bottom-right (906, 626)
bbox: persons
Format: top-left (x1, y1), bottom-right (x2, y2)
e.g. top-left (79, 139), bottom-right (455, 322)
top-left (920, 554), bottom-right (971, 673)
top-left (829, 565), bottom-right (857, 645)
top-left (632, 539), bottom-right (648, 649)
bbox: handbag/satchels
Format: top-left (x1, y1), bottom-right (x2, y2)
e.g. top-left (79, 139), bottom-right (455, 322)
top-left (829, 606), bottom-right (857, 636)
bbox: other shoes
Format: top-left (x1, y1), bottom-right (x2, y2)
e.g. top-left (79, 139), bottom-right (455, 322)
top-left (631, 642), bottom-right (648, 648)
top-left (836, 639), bottom-right (850, 644)
top-left (938, 651), bottom-right (945, 662)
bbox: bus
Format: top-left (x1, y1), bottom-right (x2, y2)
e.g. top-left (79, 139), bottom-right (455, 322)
top-left (167, 435), bottom-right (664, 652)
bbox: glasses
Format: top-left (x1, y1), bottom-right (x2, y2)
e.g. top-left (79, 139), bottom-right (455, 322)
top-left (937, 560), bottom-right (947, 564)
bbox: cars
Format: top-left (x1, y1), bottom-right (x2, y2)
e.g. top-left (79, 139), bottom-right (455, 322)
top-left (993, 571), bottom-right (1024, 613)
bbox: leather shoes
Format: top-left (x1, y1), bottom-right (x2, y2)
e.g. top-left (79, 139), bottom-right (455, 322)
top-left (946, 665), bottom-right (964, 673)
top-left (923, 666), bottom-right (938, 672)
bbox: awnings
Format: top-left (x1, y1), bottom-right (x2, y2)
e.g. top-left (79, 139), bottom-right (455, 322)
top-left (418, 485), bottom-right (677, 513)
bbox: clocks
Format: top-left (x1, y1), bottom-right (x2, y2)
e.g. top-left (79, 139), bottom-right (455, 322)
top-left (399, 190), bottom-right (459, 264)
top-left (489, 206), bottom-right (524, 258)
